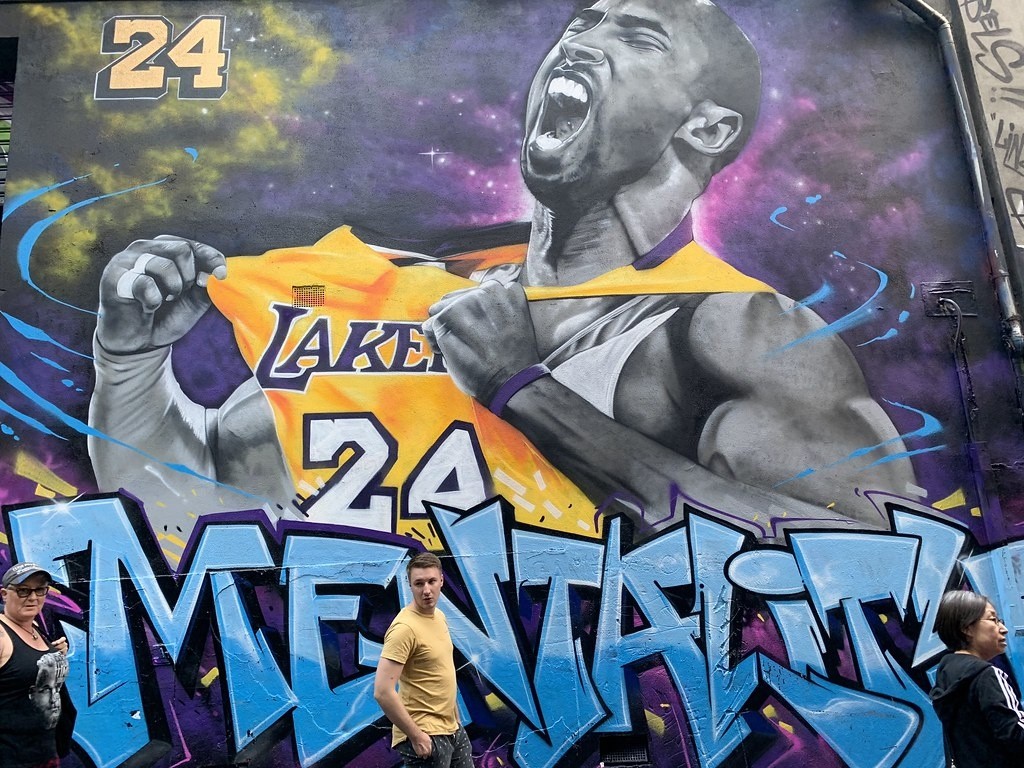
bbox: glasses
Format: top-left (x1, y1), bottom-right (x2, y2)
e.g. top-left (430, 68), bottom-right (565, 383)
top-left (978, 617), bottom-right (1004, 626)
top-left (6, 586), bottom-right (50, 598)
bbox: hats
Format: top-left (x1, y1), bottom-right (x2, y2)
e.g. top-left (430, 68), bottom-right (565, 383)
top-left (2, 562), bottom-right (53, 588)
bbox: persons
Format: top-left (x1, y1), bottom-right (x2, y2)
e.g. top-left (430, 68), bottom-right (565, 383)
top-left (930, 591), bottom-right (1024, 768)
top-left (374, 553), bottom-right (475, 768)
top-left (0, 563), bottom-right (68, 768)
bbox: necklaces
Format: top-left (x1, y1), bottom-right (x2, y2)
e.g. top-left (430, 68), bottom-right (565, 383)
top-left (2, 611), bottom-right (38, 639)
